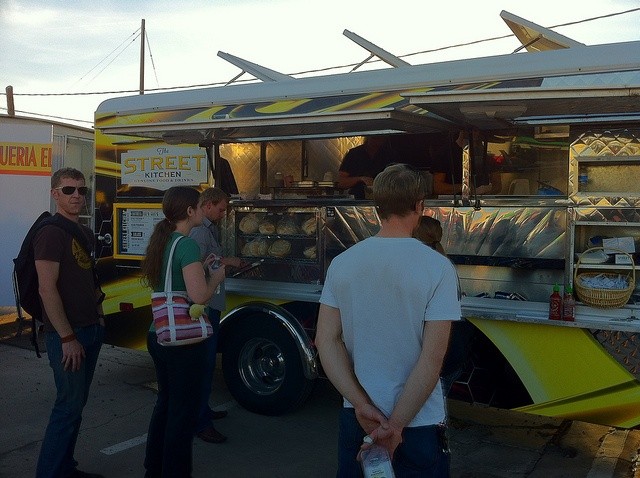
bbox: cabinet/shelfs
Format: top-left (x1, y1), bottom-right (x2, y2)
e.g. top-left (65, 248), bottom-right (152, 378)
top-left (225, 206), bottom-right (321, 264)
top-left (567, 126), bottom-right (640, 307)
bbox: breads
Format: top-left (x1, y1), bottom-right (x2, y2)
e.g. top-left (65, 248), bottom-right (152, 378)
top-left (259, 222), bottom-right (275, 234)
top-left (276, 224), bottom-right (299, 234)
top-left (302, 217), bottom-right (317, 235)
top-left (268, 239), bottom-right (292, 257)
top-left (303, 245), bottom-right (317, 259)
top-left (258, 242), bottom-right (267, 256)
top-left (238, 213), bottom-right (263, 233)
top-left (240, 240), bottom-right (258, 255)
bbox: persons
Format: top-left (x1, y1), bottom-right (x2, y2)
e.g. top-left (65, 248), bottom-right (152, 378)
top-left (413, 216), bottom-right (445, 254)
top-left (315, 163), bottom-right (461, 474)
top-left (433, 126), bottom-right (492, 194)
top-left (139, 187), bottom-right (225, 474)
top-left (32, 166), bottom-right (104, 472)
top-left (340, 135), bottom-right (404, 199)
top-left (190, 187), bottom-right (227, 443)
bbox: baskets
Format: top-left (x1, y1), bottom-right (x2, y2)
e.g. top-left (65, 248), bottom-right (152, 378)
top-left (574, 246), bottom-right (636, 309)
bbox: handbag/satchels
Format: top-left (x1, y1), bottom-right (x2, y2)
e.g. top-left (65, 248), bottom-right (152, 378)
top-left (149, 292), bottom-right (215, 347)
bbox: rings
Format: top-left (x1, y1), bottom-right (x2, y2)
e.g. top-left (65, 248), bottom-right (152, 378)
top-left (363, 434), bottom-right (374, 445)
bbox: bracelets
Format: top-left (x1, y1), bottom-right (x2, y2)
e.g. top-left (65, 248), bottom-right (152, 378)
top-left (61, 335), bottom-right (76, 343)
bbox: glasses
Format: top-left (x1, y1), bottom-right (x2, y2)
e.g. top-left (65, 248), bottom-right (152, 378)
top-left (56, 185), bottom-right (88, 197)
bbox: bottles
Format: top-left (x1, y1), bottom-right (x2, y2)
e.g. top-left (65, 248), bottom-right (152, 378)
top-left (562, 288), bottom-right (576, 321)
top-left (549, 286), bottom-right (563, 320)
top-left (359, 436), bottom-right (395, 474)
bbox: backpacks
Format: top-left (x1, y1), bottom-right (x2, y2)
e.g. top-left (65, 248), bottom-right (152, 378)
top-left (12, 211), bottom-right (87, 326)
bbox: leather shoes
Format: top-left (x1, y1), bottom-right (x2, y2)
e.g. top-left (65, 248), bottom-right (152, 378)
top-left (200, 427), bottom-right (229, 444)
top-left (210, 410), bottom-right (229, 419)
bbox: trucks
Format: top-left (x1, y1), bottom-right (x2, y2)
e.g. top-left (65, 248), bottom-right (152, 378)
top-left (88, 10), bottom-right (640, 429)
